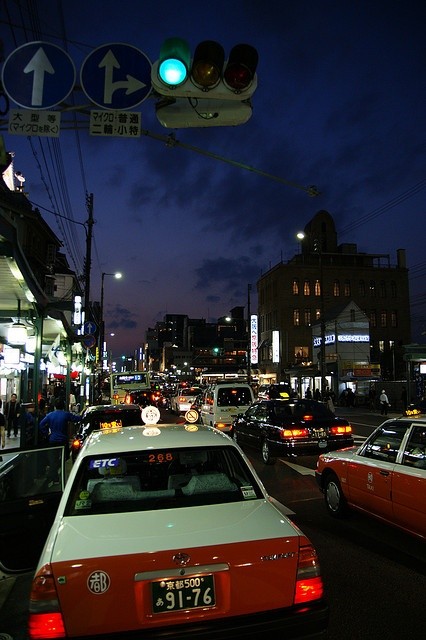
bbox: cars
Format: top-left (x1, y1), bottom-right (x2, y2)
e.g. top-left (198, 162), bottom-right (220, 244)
top-left (124, 390), bottom-right (158, 412)
top-left (0, 405), bottom-right (330, 640)
top-left (314, 403), bottom-right (425, 542)
top-left (71, 404), bottom-right (144, 464)
top-left (230, 397), bottom-right (354, 464)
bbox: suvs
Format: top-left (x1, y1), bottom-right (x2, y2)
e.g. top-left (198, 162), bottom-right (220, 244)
top-left (170, 388), bottom-right (202, 415)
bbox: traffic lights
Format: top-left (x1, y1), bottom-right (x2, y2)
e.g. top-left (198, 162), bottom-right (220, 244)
top-left (150, 37), bottom-right (259, 94)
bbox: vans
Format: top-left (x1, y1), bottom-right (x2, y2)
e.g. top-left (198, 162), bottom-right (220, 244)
top-left (258, 384), bottom-right (290, 400)
top-left (201, 383), bottom-right (254, 433)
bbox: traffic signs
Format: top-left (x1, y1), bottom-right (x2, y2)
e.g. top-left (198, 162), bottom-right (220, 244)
top-left (89, 109), bottom-right (140, 137)
top-left (8, 108), bottom-right (60, 136)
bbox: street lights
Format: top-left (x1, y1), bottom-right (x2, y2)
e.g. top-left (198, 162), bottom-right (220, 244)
top-left (296, 232), bottom-right (328, 401)
top-left (97, 273), bottom-right (121, 356)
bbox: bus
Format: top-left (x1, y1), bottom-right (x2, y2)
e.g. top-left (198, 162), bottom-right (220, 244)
top-left (109, 371), bottom-right (151, 405)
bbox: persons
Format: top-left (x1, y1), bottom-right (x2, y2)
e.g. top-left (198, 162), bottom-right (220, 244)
top-left (306, 386), bottom-right (354, 407)
top-left (3, 394), bottom-right (21, 439)
top-left (380, 390), bottom-right (391, 416)
top-left (401, 386), bottom-right (407, 413)
top-left (39, 399), bottom-right (83, 485)
top-left (0, 399), bottom-right (7, 463)
